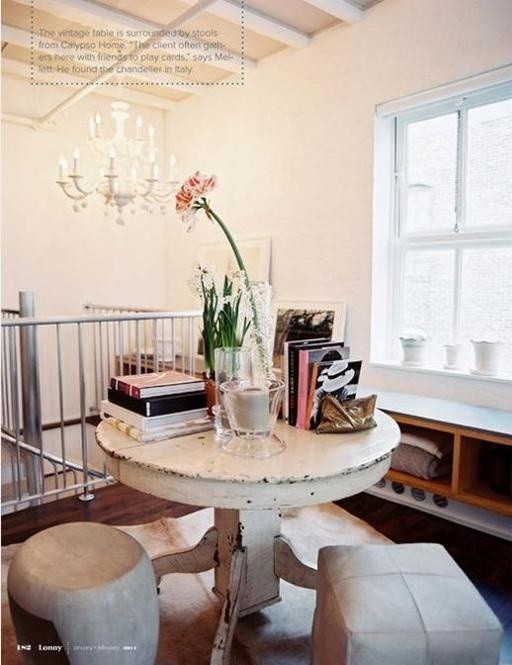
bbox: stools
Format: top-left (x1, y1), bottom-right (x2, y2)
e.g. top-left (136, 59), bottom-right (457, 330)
top-left (7, 521), bottom-right (160, 665)
top-left (312, 544), bottom-right (502, 665)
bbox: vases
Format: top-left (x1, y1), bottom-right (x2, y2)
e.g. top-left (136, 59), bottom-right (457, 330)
top-left (399, 337), bottom-right (428, 367)
top-left (239, 282), bottom-right (272, 378)
top-left (445, 344), bottom-right (462, 369)
top-left (470, 339), bottom-right (507, 376)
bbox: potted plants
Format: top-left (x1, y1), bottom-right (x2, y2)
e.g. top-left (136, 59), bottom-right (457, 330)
top-left (189, 264), bottom-right (253, 429)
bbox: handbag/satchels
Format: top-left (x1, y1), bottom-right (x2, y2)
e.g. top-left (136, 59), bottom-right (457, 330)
top-left (316, 392), bottom-right (378, 434)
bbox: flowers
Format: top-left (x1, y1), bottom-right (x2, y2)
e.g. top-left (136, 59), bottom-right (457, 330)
top-left (176, 172), bottom-right (270, 376)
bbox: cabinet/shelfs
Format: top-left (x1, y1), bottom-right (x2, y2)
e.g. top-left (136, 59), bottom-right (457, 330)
top-left (364, 409), bottom-right (512, 542)
top-left (115, 356), bottom-right (206, 378)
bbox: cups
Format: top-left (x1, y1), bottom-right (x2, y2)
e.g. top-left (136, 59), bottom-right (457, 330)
top-left (214, 346), bottom-right (256, 438)
top-left (219, 379), bottom-right (286, 457)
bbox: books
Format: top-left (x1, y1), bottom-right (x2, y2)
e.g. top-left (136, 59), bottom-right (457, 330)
top-left (100, 370), bottom-right (213, 445)
top-left (273, 308), bottom-right (363, 430)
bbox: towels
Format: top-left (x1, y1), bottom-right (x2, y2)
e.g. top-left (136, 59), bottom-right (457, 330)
top-left (391, 431), bottom-right (453, 481)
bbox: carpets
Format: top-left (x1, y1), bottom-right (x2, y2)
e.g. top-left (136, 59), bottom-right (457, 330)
top-left (1, 503), bottom-right (395, 665)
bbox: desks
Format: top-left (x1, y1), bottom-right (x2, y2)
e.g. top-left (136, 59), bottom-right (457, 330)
top-left (95, 410), bottom-right (401, 665)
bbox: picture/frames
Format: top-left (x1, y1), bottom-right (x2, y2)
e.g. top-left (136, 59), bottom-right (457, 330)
top-left (271, 300), bottom-right (347, 374)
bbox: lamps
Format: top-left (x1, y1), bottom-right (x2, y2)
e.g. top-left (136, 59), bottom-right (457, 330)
top-left (57, 101), bottom-right (180, 226)
